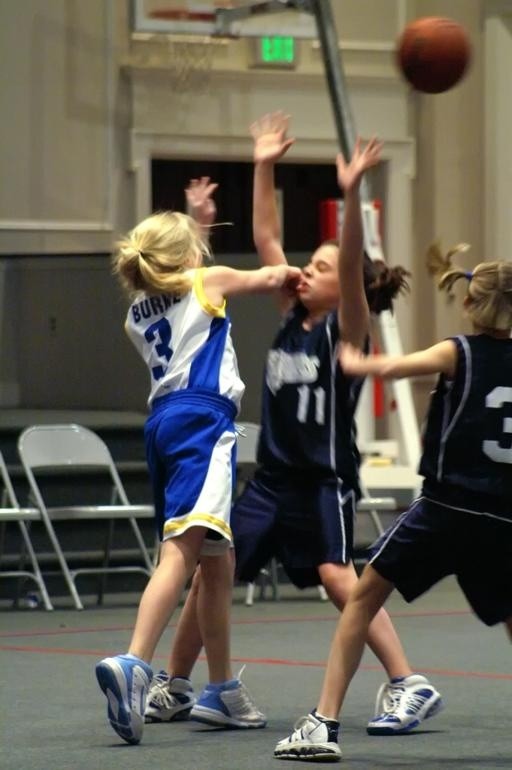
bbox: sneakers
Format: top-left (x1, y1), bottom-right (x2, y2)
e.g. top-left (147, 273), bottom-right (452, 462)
top-left (94, 653), bottom-right (152, 746)
top-left (190, 678), bottom-right (268, 730)
top-left (275, 706), bottom-right (344, 764)
top-left (144, 670), bottom-right (196, 725)
top-left (366, 670), bottom-right (445, 737)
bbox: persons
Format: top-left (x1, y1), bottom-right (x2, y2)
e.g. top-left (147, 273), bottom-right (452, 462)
top-left (275, 239), bottom-right (511, 764)
top-left (142, 108), bottom-right (443, 739)
top-left (94, 173), bottom-right (305, 750)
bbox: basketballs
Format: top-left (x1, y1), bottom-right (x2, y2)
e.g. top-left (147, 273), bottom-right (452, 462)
top-left (397, 17), bottom-right (472, 95)
